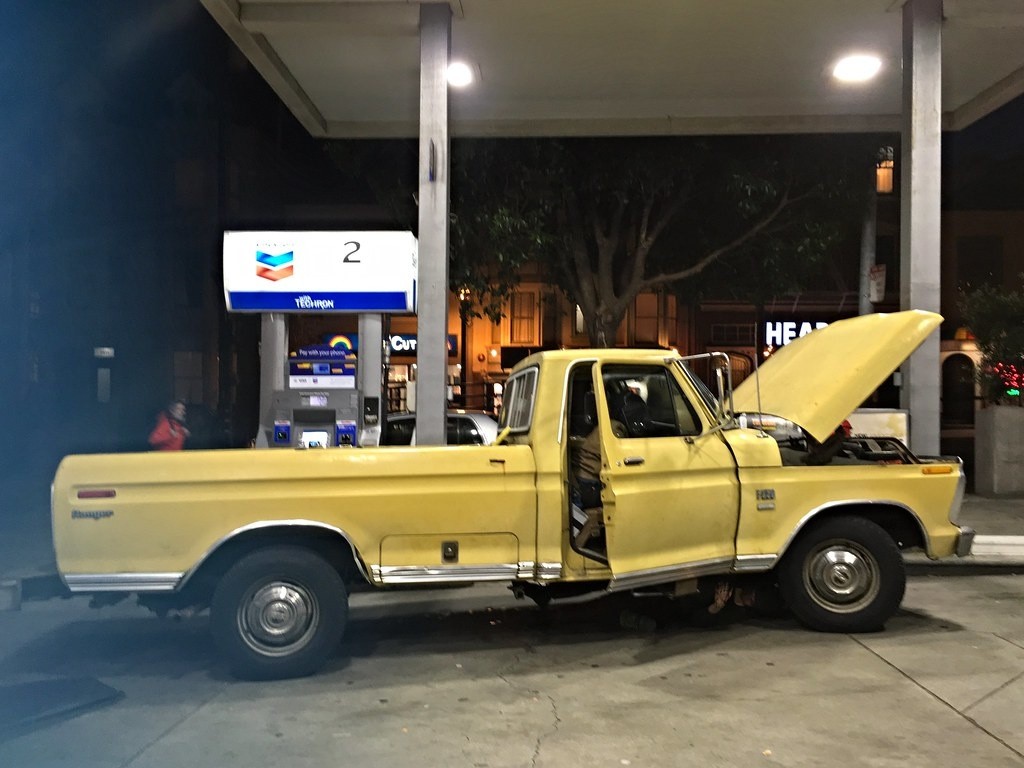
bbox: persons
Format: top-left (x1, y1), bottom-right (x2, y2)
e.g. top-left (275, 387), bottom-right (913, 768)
top-left (510, 580), bottom-right (758, 627)
top-left (580, 392), bottom-right (650, 510)
top-left (148, 401), bottom-right (192, 451)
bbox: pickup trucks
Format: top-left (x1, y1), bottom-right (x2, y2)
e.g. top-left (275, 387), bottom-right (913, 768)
top-left (0, 307), bottom-right (977, 680)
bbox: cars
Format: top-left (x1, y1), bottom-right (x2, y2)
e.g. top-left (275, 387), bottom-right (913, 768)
top-left (383, 409), bottom-right (500, 447)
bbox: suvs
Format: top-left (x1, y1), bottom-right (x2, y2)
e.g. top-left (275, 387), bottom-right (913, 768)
top-left (623, 382), bottom-right (805, 443)
